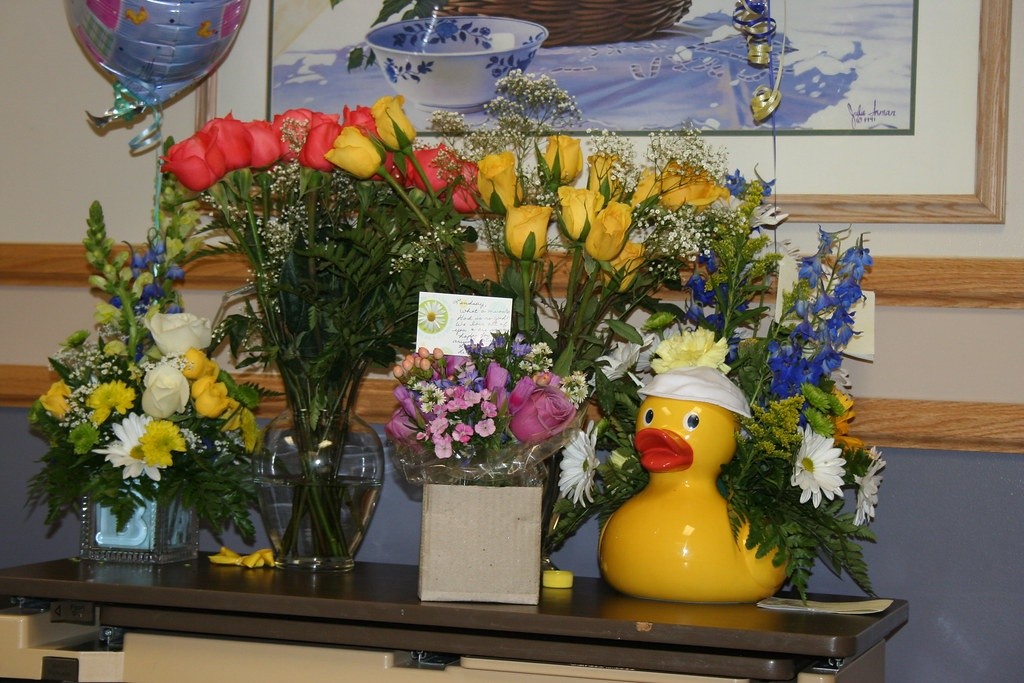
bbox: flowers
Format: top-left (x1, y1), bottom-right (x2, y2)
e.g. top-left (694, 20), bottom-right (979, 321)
top-left (13, 66), bottom-right (891, 606)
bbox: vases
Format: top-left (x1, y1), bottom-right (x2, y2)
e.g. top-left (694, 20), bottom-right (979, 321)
top-left (255, 406), bottom-right (385, 572)
top-left (80, 470), bottom-right (201, 564)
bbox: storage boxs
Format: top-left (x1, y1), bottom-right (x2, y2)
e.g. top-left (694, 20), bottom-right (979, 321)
top-left (418, 484), bottom-right (545, 605)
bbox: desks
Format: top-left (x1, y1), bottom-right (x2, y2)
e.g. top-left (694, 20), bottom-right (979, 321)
top-left (0, 549), bottom-right (911, 683)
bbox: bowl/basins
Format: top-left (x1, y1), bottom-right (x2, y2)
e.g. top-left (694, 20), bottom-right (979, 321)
top-left (364, 17), bottom-right (548, 114)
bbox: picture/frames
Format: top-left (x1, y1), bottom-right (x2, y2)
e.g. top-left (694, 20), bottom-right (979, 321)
top-left (197, 0), bottom-right (1012, 225)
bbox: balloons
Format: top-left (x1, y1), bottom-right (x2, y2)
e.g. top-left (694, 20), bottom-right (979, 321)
top-left (69, 0), bottom-right (249, 132)
